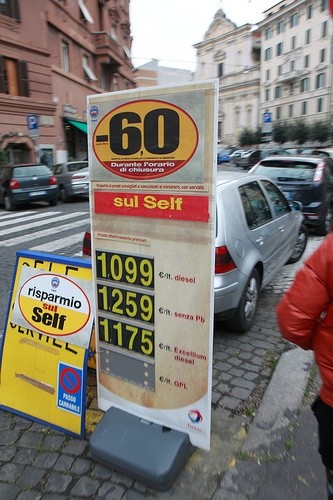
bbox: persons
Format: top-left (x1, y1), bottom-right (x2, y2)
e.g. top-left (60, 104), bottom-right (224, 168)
top-left (275, 227), bottom-right (333, 498)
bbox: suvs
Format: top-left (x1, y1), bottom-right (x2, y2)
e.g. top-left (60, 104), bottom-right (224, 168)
top-left (0, 163), bottom-right (59, 211)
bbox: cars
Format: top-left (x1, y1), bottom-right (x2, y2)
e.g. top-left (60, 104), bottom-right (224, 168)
top-left (49, 160), bottom-right (89, 204)
top-left (216, 139), bottom-right (333, 170)
top-left (83, 171), bottom-right (308, 335)
top-left (247, 154), bottom-right (333, 237)
